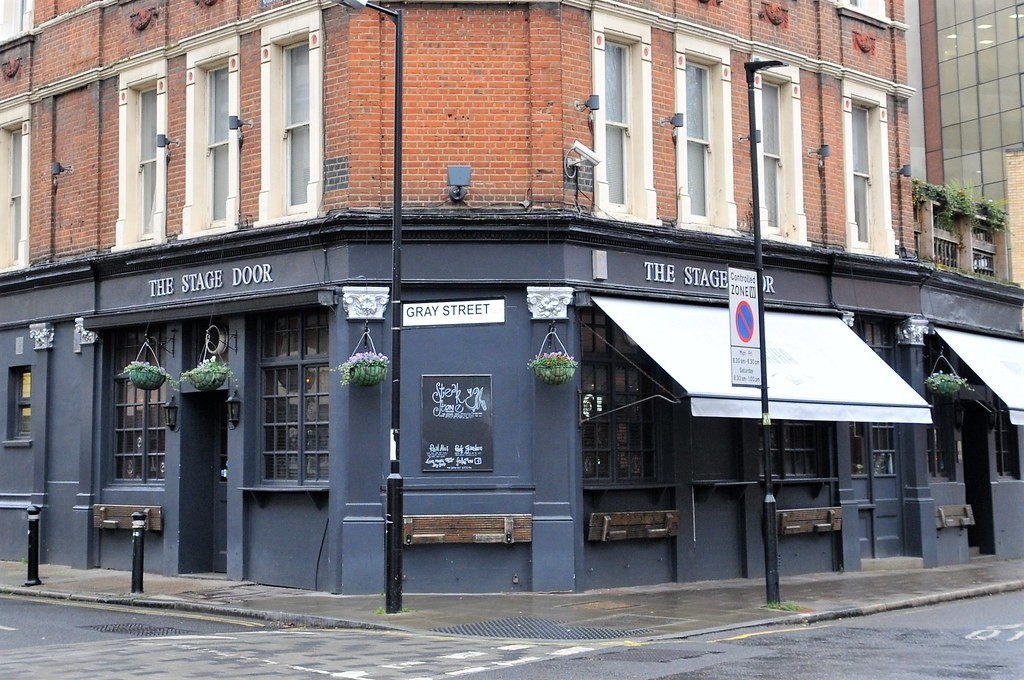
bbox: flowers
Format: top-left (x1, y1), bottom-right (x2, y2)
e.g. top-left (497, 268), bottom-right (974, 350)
top-left (122, 359), bottom-right (177, 391)
top-left (328, 351), bottom-right (389, 388)
top-left (526, 351), bottom-right (580, 370)
top-left (178, 355), bottom-right (239, 391)
top-left (923, 370), bottom-right (976, 393)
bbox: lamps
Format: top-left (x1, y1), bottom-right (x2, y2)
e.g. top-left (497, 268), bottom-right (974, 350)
top-left (890, 164), bottom-right (911, 178)
top-left (659, 112), bottom-right (685, 128)
top-left (807, 144), bottom-right (831, 158)
top-left (574, 94), bottom-right (600, 112)
top-left (225, 391), bottom-right (241, 431)
top-left (157, 134), bottom-right (181, 148)
top-left (446, 165), bottom-right (473, 201)
top-left (51, 161), bottom-right (75, 175)
top-left (229, 115), bottom-right (254, 130)
top-left (738, 129), bottom-right (762, 144)
top-left (160, 394), bottom-right (180, 433)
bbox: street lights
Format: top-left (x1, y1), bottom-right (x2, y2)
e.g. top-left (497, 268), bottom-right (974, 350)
top-left (327, 0), bottom-right (404, 615)
top-left (743, 59), bottom-right (791, 611)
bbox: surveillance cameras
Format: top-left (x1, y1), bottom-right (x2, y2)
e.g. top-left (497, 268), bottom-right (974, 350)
top-left (574, 140), bottom-right (601, 166)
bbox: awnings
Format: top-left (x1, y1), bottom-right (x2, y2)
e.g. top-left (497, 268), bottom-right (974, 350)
top-left (934, 327), bottom-right (1024, 425)
top-left (589, 296), bottom-right (933, 425)
